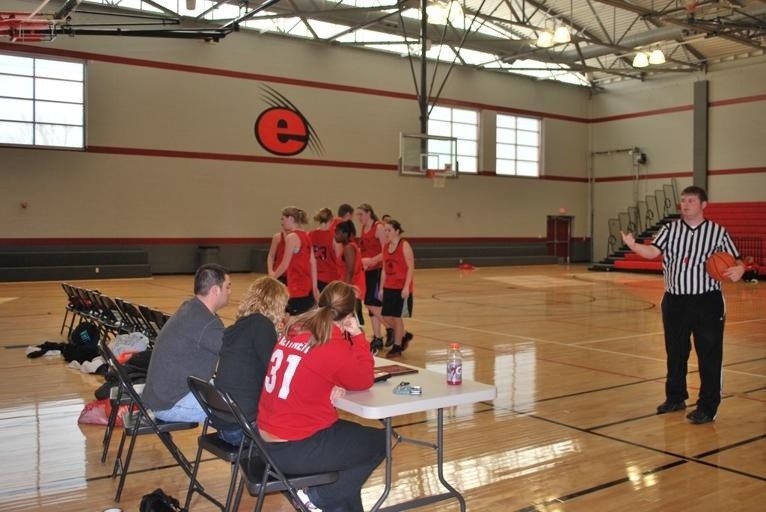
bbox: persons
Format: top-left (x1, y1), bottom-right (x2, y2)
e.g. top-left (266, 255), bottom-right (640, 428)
top-left (213, 276), bottom-right (290, 447)
top-left (255, 279), bottom-right (386, 512)
top-left (620, 186), bottom-right (746, 424)
top-left (141, 262), bottom-right (232, 424)
top-left (742, 256), bottom-right (760, 284)
top-left (267, 203), bottom-right (415, 359)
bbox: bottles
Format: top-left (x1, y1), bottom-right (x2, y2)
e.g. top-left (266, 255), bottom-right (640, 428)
top-left (447, 342), bottom-right (464, 386)
top-left (458, 257), bottom-right (463, 265)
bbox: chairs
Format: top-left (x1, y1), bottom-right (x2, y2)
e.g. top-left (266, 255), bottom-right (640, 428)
top-left (184, 374), bottom-right (340, 512)
top-left (60, 282), bottom-right (174, 352)
top-left (95, 338), bottom-right (205, 503)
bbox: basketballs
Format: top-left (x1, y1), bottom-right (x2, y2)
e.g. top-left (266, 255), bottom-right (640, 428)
top-left (706, 252), bottom-right (737, 281)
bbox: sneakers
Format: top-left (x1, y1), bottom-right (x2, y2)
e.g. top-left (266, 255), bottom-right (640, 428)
top-left (385, 328), bottom-right (395, 347)
top-left (292, 490), bottom-right (322, 512)
top-left (657, 400), bottom-right (686, 413)
top-left (370, 335), bottom-right (383, 356)
top-left (686, 410), bottom-right (712, 423)
top-left (401, 333), bottom-right (413, 351)
top-left (386, 344), bottom-right (401, 358)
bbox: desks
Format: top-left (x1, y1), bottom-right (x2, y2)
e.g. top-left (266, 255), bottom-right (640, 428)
top-left (331, 353), bottom-right (497, 512)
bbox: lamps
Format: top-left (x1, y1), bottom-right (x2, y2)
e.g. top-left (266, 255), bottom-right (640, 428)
top-left (536, 13), bottom-right (572, 48)
top-left (631, 42), bottom-right (667, 69)
top-left (425, 0), bottom-right (465, 26)
